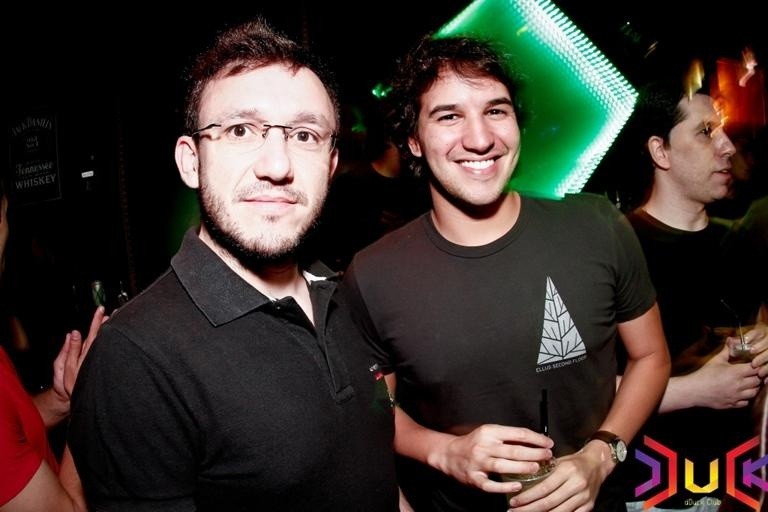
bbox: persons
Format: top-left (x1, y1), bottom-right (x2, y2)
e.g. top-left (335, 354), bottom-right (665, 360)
top-left (121, 0), bottom-right (768, 283)
top-left (0, 172), bottom-right (121, 511)
top-left (69, 18), bottom-right (403, 511)
top-left (334, 34), bottom-right (674, 511)
top-left (612, 77), bottom-right (768, 512)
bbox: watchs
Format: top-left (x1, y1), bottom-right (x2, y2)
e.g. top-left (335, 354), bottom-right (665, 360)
top-left (583, 428), bottom-right (630, 466)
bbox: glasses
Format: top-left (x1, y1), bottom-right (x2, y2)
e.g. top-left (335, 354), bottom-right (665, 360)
top-left (189, 119), bottom-right (338, 151)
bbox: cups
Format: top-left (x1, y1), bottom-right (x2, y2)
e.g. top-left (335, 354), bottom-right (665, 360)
top-left (727, 343), bottom-right (759, 364)
top-left (497, 457), bottom-right (560, 500)
